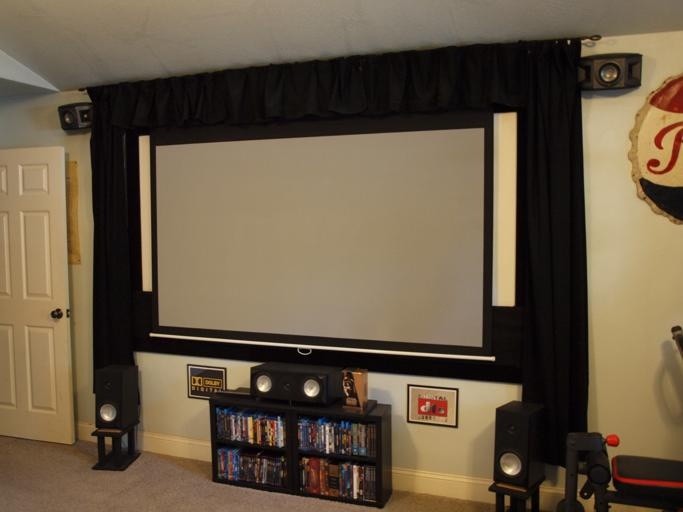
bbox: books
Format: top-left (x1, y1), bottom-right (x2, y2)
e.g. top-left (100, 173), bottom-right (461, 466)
top-left (215, 405), bottom-right (377, 502)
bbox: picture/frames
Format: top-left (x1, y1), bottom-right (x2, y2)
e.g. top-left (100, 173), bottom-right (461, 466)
top-left (186, 364), bottom-right (227, 400)
top-left (406, 383), bottom-right (458, 427)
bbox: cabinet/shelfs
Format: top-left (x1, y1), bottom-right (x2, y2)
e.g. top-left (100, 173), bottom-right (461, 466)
top-left (208, 389), bottom-right (392, 509)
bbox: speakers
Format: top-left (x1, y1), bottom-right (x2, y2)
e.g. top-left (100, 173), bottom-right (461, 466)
top-left (248, 361), bottom-right (343, 407)
top-left (93, 363), bottom-right (139, 430)
top-left (57, 100), bottom-right (90, 132)
top-left (581, 50), bottom-right (642, 92)
top-left (492, 399), bottom-right (548, 491)
